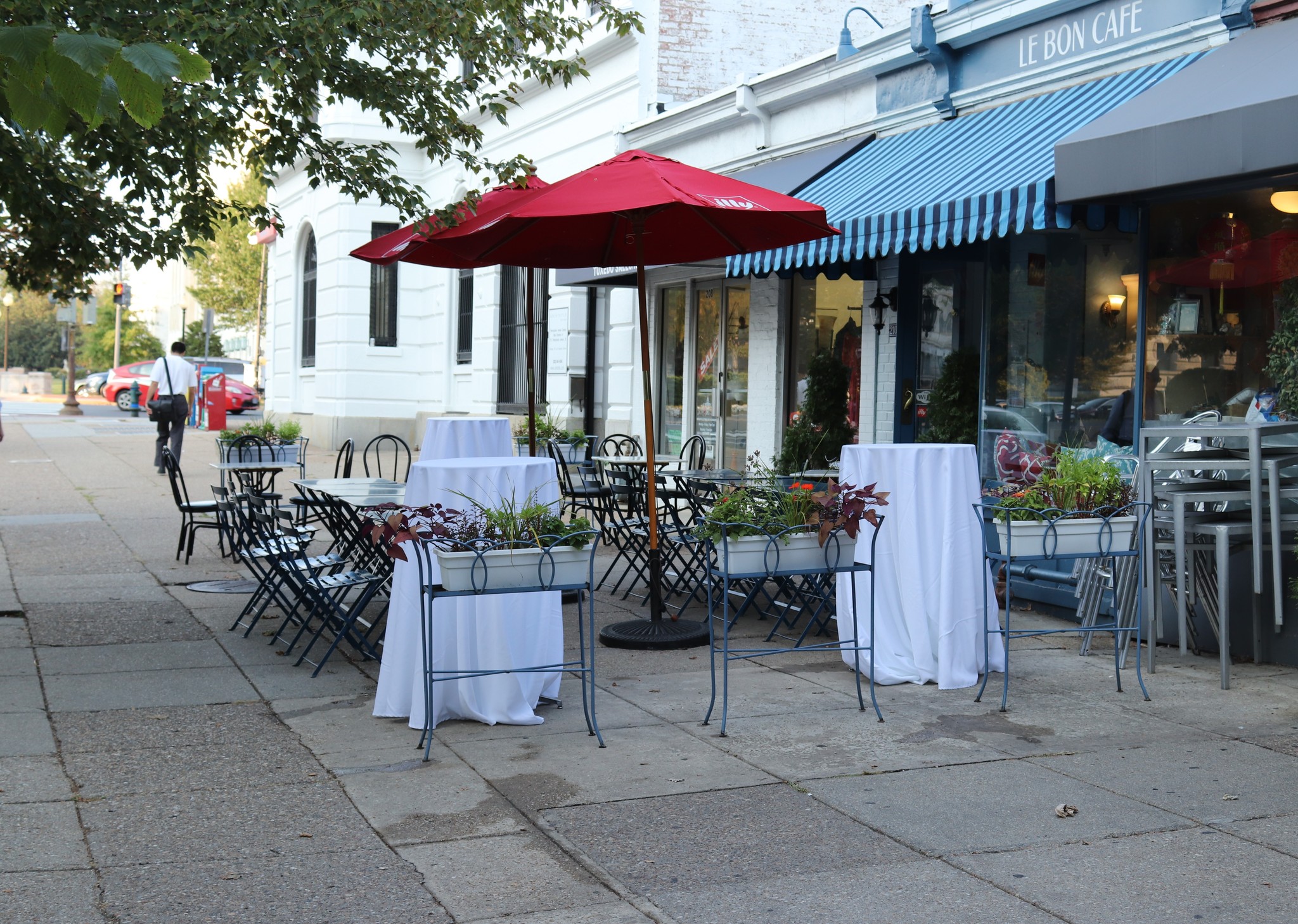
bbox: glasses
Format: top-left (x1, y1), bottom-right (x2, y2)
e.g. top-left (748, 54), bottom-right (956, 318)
top-left (1146, 376), bottom-right (1162, 382)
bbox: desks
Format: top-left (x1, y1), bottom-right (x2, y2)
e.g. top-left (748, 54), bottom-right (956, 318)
top-left (649, 468), bottom-right (783, 474)
top-left (338, 496), bottom-right (407, 510)
top-left (1138, 421), bottom-right (1298, 698)
top-left (286, 471), bottom-right (399, 485)
top-left (298, 481), bottom-right (407, 493)
top-left (206, 453), bottom-right (304, 479)
top-left (373, 457), bottom-right (567, 728)
top-left (589, 448), bottom-right (687, 467)
top-left (788, 464), bottom-right (840, 482)
top-left (674, 471), bottom-right (796, 481)
top-left (322, 489), bottom-right (414, 501)
top-left (709, 478), bottom-right (819, 486)
top-left (836, 442), bottom-right (1006, 691)
top-left (417, 416), bottom-right (515, 459)
top-left (758, 485), bottom-right (843, 500)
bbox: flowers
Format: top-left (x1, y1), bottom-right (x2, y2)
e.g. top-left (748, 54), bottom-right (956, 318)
top-left (1008, 484), bottom-right (1031, 498)
top-left (788, 477), bottom-right (814, 502)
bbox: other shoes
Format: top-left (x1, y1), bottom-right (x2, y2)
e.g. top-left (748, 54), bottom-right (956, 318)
top-left (158, 466), bottom-right (165, 473)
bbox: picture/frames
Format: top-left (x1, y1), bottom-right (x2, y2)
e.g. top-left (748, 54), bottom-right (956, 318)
top-left (1175, 299), bottom-right (1200, 334)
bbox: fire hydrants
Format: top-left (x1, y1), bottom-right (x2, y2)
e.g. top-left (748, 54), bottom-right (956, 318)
top-left (127, 381), bottom-right (142, 416)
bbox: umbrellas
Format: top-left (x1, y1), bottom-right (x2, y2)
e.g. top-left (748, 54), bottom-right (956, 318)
top-left (413, 150), bottom-right (845, 651)
top-left (349, 177), bottom-right (586, 604)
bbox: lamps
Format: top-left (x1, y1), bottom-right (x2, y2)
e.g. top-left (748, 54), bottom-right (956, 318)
top-left (1267, 185), bottom-right (1298, 220)
top-left (1098, 287), bottom-right (1128, 330)
top-left (871, 283), bottom-right (902, 337)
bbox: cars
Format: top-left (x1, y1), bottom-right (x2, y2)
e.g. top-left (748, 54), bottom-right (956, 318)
top-left (104, 360), bottom-right (258, 414)
top-left (982, 397), bottom-right (1117, 482)
top-left (73, 371), bottom-right (109, 398)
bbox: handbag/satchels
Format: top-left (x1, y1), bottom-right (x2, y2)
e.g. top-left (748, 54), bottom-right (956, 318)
top-left (148, 399), bottom-right (173, 421)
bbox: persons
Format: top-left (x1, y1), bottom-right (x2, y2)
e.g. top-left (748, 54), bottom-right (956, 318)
top-left (145, 342), bottom-right (199, 477)
top-left (1101, 371), bottom-right (1161, 447)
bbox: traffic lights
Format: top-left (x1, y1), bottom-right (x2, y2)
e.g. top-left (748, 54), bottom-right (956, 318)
top-left (113, 283), bottom-right (130, 307)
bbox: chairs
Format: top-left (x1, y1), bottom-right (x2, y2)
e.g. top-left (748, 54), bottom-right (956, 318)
top-left (532, 434), bottom-right (847, 643)
top-left (152, 433), bottom-right (414, 691)
top-left (1040, 409), bottom-right (1231, 687)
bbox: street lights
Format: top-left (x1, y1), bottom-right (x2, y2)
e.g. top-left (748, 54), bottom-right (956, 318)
top-left (3, 293), bottom-right (13, 371)
top-left (180, 300), bottom-right (188, 344)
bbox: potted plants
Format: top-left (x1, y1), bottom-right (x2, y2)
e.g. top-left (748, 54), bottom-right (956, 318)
top-left (692, 463), bottom-right (890, 573)
top-left (372, 495), bottom-right (599, 590)
top-left (214, 416), bottom-right (304, 469)
top-left (512, 412), bottom-right (588, 461)
top-left (981, 440), bottom-right (1143, 558)
